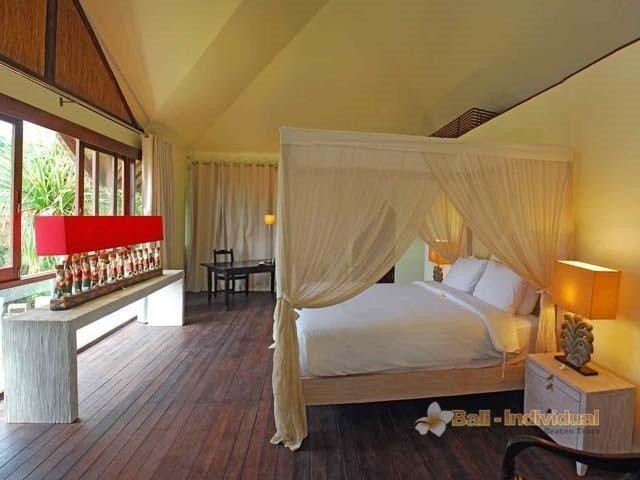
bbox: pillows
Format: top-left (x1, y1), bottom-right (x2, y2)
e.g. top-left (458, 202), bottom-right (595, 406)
top-left (442, 254), bottom-right (540, 316)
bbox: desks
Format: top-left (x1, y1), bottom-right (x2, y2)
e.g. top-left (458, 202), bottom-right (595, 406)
top-left (199, 261), bottom-right (273, 310)
top-left (5, 270), bottom-right (186, 422)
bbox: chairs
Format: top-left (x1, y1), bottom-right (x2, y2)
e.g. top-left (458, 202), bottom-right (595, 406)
top-left (213, 250), bottom-right (248, 297)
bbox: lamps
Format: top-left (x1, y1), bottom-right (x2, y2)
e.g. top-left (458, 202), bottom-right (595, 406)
top-left (262, 213), bottom-right (277, 232)
top-left (427, 240), bottom-right (451, 280)
top-left (550, 259), bottom-right (623, 377)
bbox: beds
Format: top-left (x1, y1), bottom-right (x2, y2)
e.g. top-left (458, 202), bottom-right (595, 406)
top-left (275, 283), bottom-right (555, 445)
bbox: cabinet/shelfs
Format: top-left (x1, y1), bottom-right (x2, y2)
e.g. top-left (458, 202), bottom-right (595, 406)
top-left (524, 354), bottom-right (634, 477)
